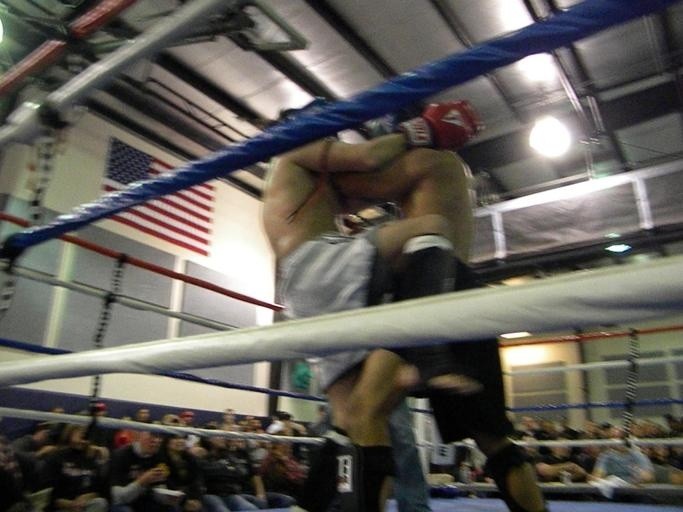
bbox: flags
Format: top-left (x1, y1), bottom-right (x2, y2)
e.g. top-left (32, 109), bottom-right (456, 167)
top-left (93, 135), bottom-right (219, 258)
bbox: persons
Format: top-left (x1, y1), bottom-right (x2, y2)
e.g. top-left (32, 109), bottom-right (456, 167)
top-left (340, 100), bottom-right (548, 511)
top-left (260, 96), bottom-right (479, 512)
top-left (0, 399), bottom-right (682, 511)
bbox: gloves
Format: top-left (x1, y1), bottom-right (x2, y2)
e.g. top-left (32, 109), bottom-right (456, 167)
top-left (394, 100), bottom-right (482, 149)
